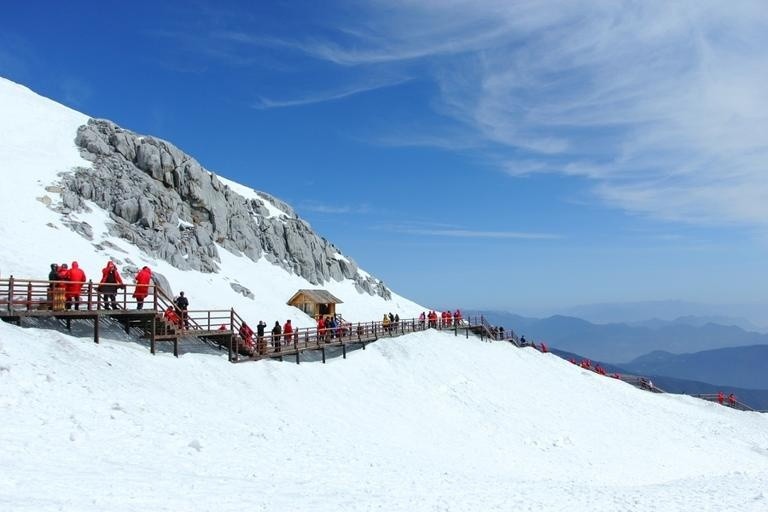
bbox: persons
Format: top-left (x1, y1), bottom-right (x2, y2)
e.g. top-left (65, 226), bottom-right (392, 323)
top-left (271, 321), bottom-right (282, 353)
top-left (65, 260), bottom-right (87, 312)
top-left (381, 308), bottom-right (462, 334)
top-left (97, 262), bottom-right (125, 311)
top-left (239, 321), bottom-right (252, 349)
top-left (718, 389), bottom-right (725, 406)
top-left (52, 263), bottom-right (69, 311)
top-left (330, 317), bottom-right (338, 338)
top-left (164, 306), bottom-right (178, 325)
top-left (283, 319), bottom-right (294, 346)
top-left (318, 315), bottom-right (326, 342)
top-left (218, 324), bottom-right (227, 330)
top-left (728, 392), bottom-right (735, 407)
top-left (132, 266), bottom-right (152, 311)
top-left (326, 317), bottom-right (331, 343)
top-left (173, 290), bottom-right (189, 332)
top-left (257, 320), bottom-right (267, 354)
top-left (490, 324), bottom-right (655, 389)
top-left (49, 263), bottom-right (70, 310)
